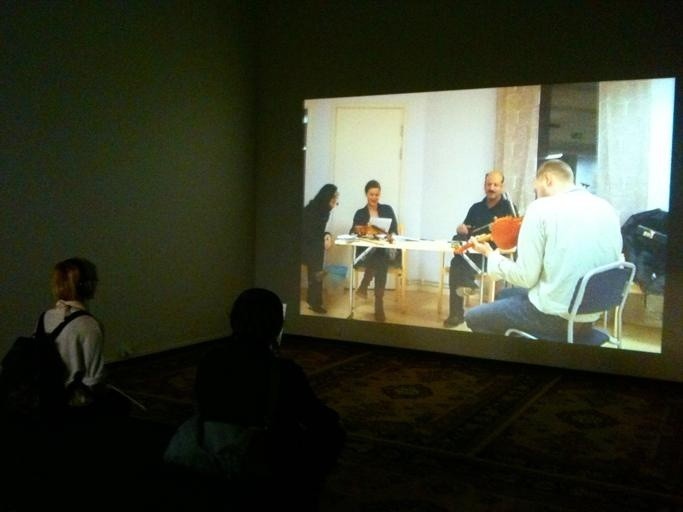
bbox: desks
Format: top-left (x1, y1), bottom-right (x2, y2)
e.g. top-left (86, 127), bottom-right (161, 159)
top-left (332, 233), bottom-right (490, 317)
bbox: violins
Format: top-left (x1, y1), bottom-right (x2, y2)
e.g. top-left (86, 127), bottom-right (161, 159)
top-left (453, 216), bottom-right (524, 257)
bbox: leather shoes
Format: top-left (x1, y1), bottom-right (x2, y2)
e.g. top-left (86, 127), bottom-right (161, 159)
top-left (444, 314), bottom-right (463, 327)
top-left (309, 302), bottom-right (327, 314)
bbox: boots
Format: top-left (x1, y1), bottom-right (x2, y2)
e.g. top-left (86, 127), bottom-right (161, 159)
top-left (357, 273), bottom-right (372, 297)
top-left (374, 288), bottom-right (386, 321)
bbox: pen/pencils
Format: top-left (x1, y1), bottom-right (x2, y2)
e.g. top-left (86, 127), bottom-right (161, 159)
top-left (405, 238), bottom-right (418, 242)
top-left (420, 238), bottom-right (434, 241)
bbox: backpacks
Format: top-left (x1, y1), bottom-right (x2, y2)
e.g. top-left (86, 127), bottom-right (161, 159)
top-left (0, 309), bottom-right (95, 440)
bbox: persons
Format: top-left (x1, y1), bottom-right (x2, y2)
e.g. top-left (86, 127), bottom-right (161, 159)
top-left (301, 184), bottom-right (340, 314)
top-left (34, 256), bottom-right (114, 410)
top-left (464, 159), bottom-right (623, 338)
top-left (443, 172), bottom-right (519, 328)
top-left (194, 288), bottom-right (345, 491)
top-left (348, 180), bottom-right (403, 321)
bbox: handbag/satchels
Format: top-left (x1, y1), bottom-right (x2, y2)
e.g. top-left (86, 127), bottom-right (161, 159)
top-left (621, 210), bottom-right (666, 293)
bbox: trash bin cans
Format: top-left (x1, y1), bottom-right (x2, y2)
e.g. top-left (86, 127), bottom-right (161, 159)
top-left (324, 264), bottom-right (348, 295)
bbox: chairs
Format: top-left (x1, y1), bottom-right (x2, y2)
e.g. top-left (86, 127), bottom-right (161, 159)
top-left (351, 222), bottom-right (512, 314)
top-left (501, 259), bottom-right (637, 349)
